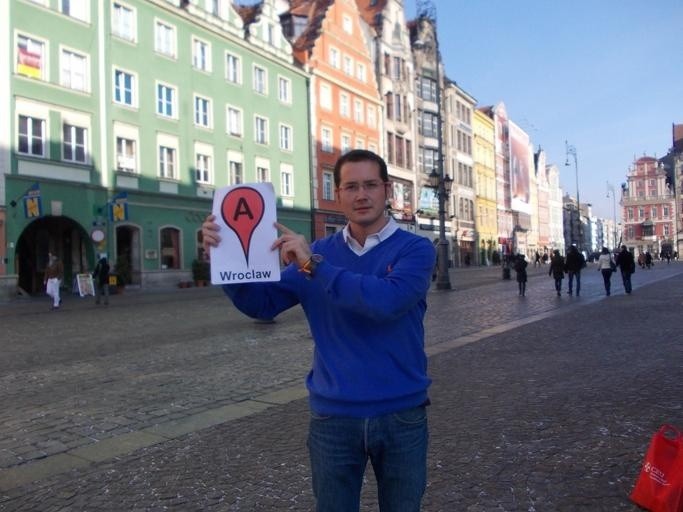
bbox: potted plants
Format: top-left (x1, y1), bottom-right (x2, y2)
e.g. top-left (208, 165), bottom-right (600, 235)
top-left (192, 258), bottom-right (206, 286)
top-left (114, 249), bottom-right (129, 295)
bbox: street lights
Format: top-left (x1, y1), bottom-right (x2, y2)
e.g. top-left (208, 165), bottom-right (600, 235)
top-left (565, 139), bottom-right (583, 255)
top-left (413, 0), bottom-right (454, 290)
top-left (606, 180), bottom-right (617, 249)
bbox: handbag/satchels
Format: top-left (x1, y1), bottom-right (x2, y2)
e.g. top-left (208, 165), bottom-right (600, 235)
top-left (628, 423), bottom-right (682, 512)
top-left (609, 255), bottom-right (616, 272)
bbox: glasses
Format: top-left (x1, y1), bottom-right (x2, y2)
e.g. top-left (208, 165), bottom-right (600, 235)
top-left (337, 180), bottom-right (387, 193)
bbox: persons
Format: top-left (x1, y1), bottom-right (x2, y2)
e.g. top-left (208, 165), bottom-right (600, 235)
top-left (92, 257), bottom-right (109, 306)
top-left (637, 249), bottom-right (678, 270)
top-left (565, 246), bottom-right (583, 297)
top-left (596, 247), bottom-right (617, 297)
top-left (548, 248), bottom-right (565, 296)
top-left (43, 253), bottom-right (65, 310)
top-left (615, 245), bottom-right (635, 295)
top-left (200, 149), bottom-right (438, 512)
top-left (500, 251), bottom-right (548, 296)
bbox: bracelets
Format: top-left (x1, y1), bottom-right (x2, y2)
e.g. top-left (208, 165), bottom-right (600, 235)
top-left (297, 259), bottom-right (311, 274)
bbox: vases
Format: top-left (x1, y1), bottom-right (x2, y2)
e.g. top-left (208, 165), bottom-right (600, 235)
top-left (179, 280), bottom-right (193, 288)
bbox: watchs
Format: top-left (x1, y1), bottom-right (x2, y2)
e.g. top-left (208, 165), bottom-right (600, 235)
top-left (304, 253), bottom-right (323, 281)
